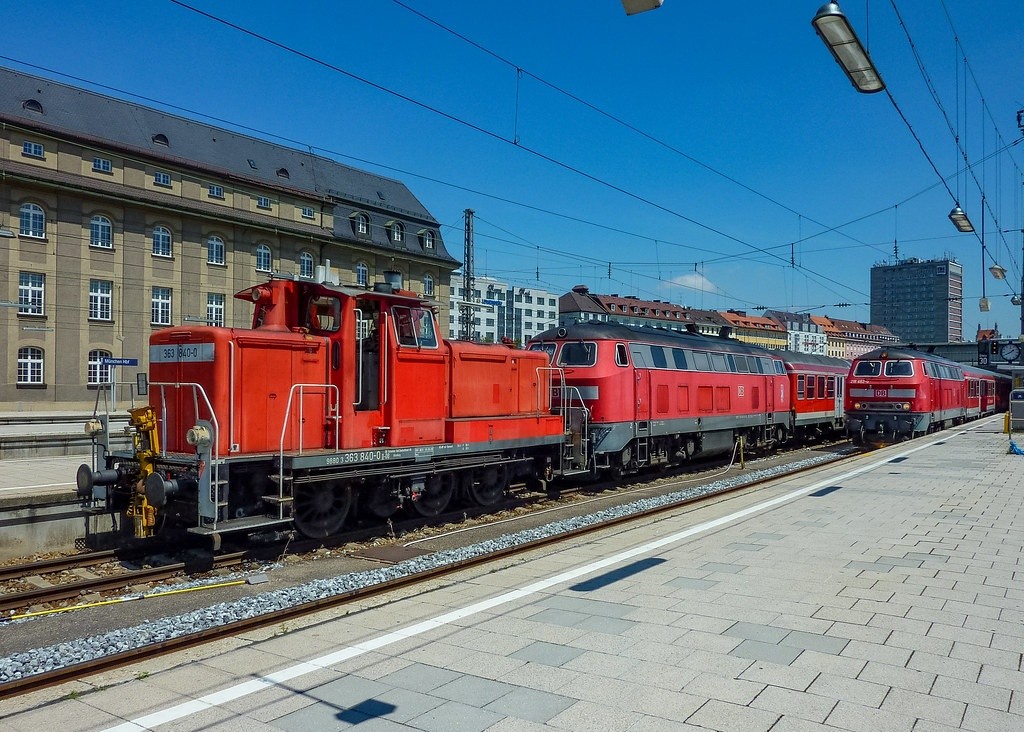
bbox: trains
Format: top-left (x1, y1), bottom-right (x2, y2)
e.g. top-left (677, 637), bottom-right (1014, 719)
top-left (843, 346), bottom-right (1011, 446)
top-left (74, 275), bottom-right (855, 574)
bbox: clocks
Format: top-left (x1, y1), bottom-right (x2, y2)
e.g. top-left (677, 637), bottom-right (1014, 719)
top-left (1001, 343), bottom-right (1020, 361)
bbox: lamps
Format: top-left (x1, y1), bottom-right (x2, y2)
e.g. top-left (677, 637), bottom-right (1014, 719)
top-left (948, 206), bottom-right (977, 232)
top-left (989, 265), bottom-right (1006, 280)
top-left (1011, 295), bottom-right (1022, 305)
top-left (812, 2), bottom-right (888, 94)
